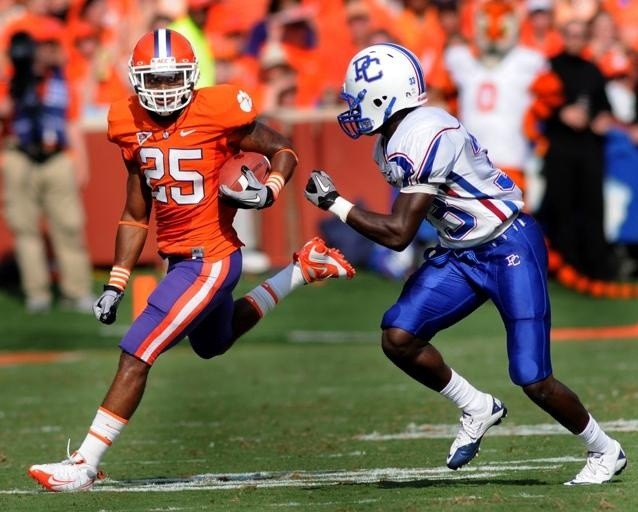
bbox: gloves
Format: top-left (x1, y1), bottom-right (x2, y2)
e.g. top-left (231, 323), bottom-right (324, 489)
top-left (219, 164), bottom-right (274, 210)
top-left (303, 170), bottom-right (341, 212)
top-left (93, 283), bottom-right (124, 326)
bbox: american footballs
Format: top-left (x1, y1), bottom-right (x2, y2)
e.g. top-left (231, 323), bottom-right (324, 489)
top-left (219, 152), bottom-right (271, 192)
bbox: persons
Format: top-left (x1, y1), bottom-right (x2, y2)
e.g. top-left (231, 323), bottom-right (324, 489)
top-left (303, 42), bottom-right (628, 487)
top-left (1, 0), bottom-right (637, 312)
top-left (29, 27), bottom-right (356, 493)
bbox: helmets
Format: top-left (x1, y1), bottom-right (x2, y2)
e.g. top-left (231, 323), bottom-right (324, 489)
top-left (336, 43), bottom-right (429, 140)
top-left (126, 28), bottom-right (201, 115)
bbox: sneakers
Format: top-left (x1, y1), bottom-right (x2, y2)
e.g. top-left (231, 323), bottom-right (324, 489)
top-left (24, 296), bottom-right (51, 315)
top-left (58, 292), bottom-right (97, 315)
top-left (27, 449), bottom-right (98, 494)
top-left (292, 236), bottom-right (357, 284)
top-left (446, 393), bottom-right (507, 470)
top-left (562, 439), bottom-right (628, 485)
